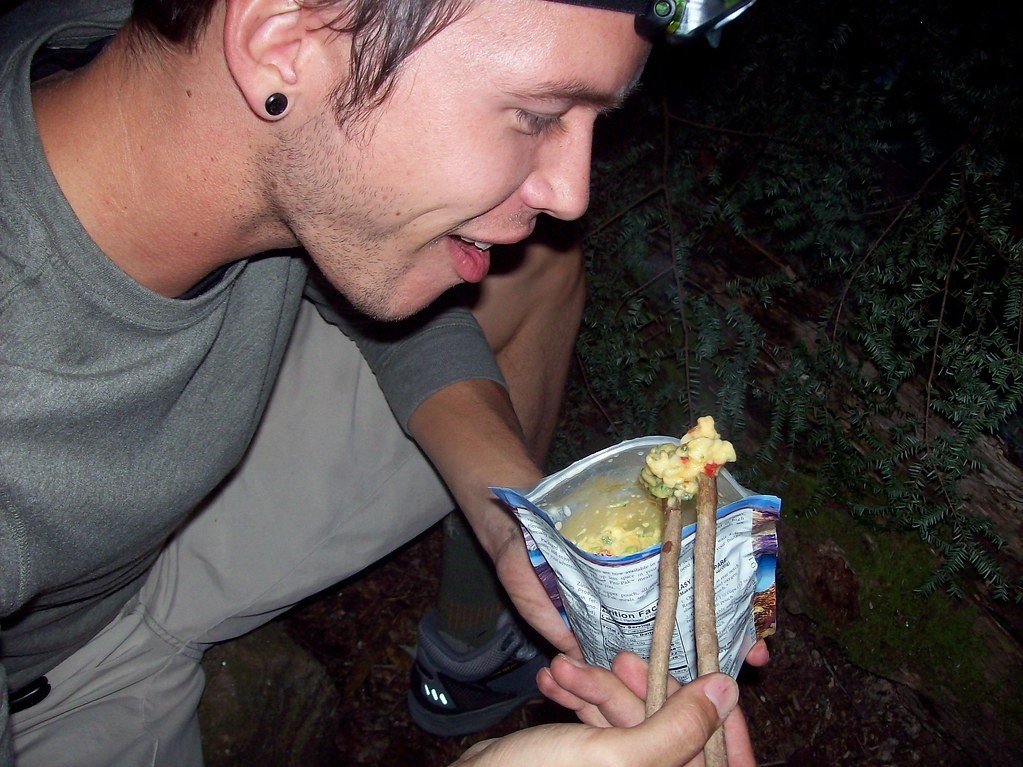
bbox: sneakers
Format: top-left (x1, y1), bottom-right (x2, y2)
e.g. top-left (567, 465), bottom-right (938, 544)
top-left (409, 612), bottom-right (563, 738)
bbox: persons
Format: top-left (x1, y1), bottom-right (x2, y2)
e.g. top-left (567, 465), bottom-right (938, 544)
top-left (0, 0), bottom-right (769, 767)
